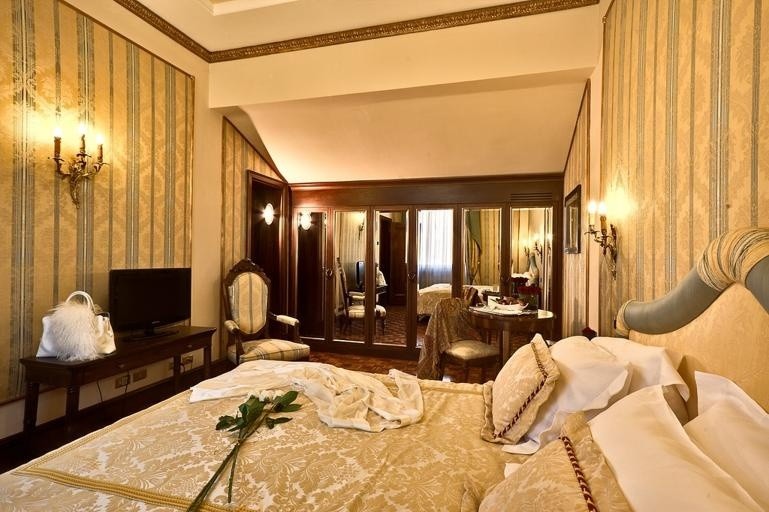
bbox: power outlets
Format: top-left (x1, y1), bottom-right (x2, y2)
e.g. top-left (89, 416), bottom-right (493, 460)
top-left (182, 355), bottom-right (193, 364)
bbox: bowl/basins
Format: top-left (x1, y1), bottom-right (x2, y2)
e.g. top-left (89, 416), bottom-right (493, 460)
top-left (492, 301), bottom-right (529, 312)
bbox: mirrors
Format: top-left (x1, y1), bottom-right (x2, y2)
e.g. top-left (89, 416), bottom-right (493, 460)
top-left (289, 200), bottom-right (563, 383)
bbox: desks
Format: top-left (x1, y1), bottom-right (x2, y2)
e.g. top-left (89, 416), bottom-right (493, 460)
top-left (20, 325), bottom-right (216, 452)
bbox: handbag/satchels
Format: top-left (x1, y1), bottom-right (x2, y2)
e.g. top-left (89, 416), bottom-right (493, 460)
top-left (36, 309), bottom-right (116, 358)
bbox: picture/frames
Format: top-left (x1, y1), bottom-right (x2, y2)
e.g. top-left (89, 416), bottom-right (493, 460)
top-left (564, 184), bottom-right (580, 254)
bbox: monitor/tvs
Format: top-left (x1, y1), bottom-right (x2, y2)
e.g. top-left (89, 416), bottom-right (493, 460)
top-left (356, 261), bottom-right (365, 291)
top-left (108, 268), bottom-right (191, 342)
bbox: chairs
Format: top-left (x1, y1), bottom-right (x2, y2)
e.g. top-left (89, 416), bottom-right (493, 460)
top-left (221, 257), bottom-right (310, 370)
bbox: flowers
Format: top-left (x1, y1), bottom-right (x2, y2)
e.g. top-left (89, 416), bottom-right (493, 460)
top-left (187, 389), bottom-right (304, 512)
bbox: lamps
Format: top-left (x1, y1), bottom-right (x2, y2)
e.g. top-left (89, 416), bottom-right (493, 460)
top-left (53, 124), bottom-right (108, 209)
top-left (583, 201), bottom-right (617, 280)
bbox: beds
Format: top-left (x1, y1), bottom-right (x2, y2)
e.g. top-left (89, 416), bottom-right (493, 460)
top-left (0, 227), bottom-right (769, 512)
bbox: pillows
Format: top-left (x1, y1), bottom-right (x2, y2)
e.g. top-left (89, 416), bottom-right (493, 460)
top-left (479, 335), bottom-right (697, 456)
top-left (478, 370), bottom-right (767, 512)
